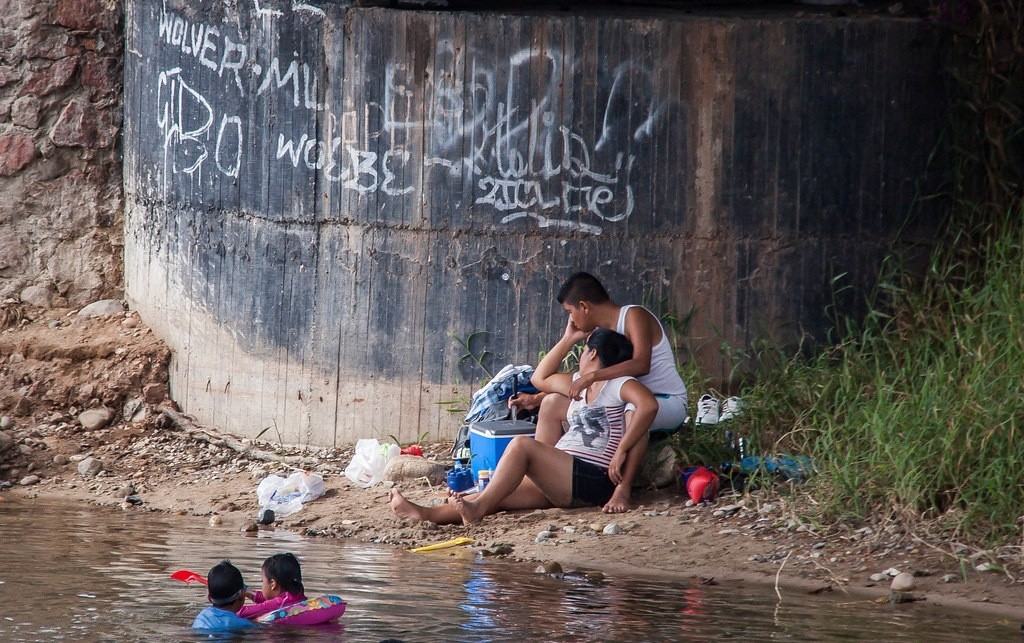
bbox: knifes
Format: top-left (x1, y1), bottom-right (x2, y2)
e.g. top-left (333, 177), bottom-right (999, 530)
top-left (512, 374), bottom-right (517, 423)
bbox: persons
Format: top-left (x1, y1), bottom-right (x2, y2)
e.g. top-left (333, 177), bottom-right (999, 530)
top-left (191, 560), bottom-right (261, 630)
top-left (236, 553), bottom-right (308, 619)
top-left (386, 314), bottom-right (661, 527)
top-left (507, 271), bottom-right (689, 514)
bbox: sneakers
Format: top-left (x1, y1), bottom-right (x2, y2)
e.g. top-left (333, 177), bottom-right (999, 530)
top-left (695, 394), bottom-right (719, 425)
top-left (719, 396), bottom-right (742, 421)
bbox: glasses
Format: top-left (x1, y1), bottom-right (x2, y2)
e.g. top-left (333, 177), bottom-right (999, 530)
top-left (243, 584), bottom-right (247, 591)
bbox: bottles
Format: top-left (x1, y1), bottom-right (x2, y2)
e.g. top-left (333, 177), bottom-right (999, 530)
top-left (736, 439), bottom-right (751, 464)
top-left (478, 470), bottom-right (489, 492)
top-left (489, 471), bottom-right (494, 480)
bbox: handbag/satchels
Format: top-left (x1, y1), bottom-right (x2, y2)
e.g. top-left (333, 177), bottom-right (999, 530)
top-left (257, 469), bottom-right (326, 517)
top-left (345, 438), bottom-right (402, 489)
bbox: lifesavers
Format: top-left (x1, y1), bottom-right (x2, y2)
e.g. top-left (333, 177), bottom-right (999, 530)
top-left (253, 594), bottom-right (347, 626)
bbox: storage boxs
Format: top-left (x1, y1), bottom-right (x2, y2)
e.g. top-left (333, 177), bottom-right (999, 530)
top-left (469, 418), bottom-right (536, 489)
